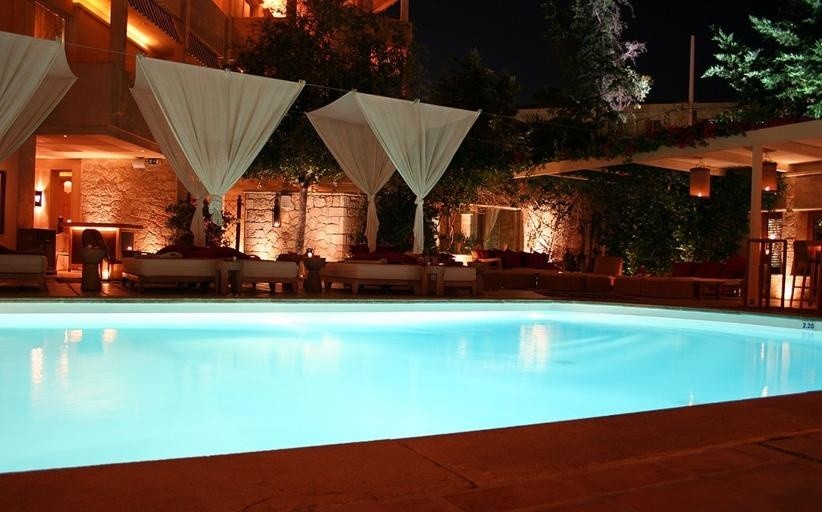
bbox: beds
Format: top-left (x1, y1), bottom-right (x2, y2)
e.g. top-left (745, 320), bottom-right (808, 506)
top-left (621, 263), bottom-right (745, 298)
top-left (234, 259), bottom-right (306, 298)
top-left (120, 256), bottom-right (226, 298)
top-left (470, 248), bottom-right (562, 290)
top-left (444, 266), bottom-right (479, 296)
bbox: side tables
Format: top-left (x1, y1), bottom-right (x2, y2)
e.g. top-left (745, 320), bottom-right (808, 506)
top-left (422, 265), bottom-right (445, 299)
top-left (213, 260), bottom-right (241, 300)
top-left (304, 255), bottom-right (326, 290)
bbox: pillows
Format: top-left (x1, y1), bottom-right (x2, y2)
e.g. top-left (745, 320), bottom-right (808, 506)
top-left (671, 255), bottom-right (746, 276)
top-left (346, 252), bottom-right (418, 264)
top-left (471, 249), bottom-right (547, 268)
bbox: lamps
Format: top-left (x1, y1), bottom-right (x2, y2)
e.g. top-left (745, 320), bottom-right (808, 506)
top-left (132, 158), bottom-right (148, 169)
top-left (63, 169), bottom-right (72, 195)
top-left (269, 193), bottom-right (282, 229)
top-left (686, 151), bottom-right (779, 197)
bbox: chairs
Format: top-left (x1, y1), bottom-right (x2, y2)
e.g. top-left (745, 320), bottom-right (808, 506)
top-left (789, 240), bottom-right (821, 310)
top-left (321, 261), bottom-right (422, 297)
top-left (544, 254), bottom-right (624, 292)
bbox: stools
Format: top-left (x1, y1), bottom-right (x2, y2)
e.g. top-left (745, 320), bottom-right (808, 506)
top-left (1, 251), bottom-right (49, 292)
